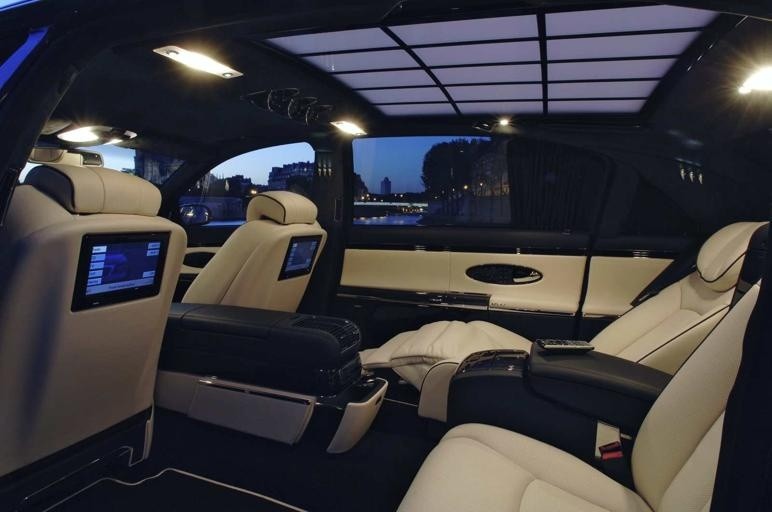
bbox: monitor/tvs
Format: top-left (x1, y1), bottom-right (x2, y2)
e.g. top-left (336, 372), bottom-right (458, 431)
top-left (278, 235), bottom-right (322, 281)
top-left (71, 231), bottom-right (170, 312)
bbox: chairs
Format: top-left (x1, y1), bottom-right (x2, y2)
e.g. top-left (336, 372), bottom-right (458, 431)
top-left (358, 219), bottom-right (771, 491)
top-left (393, 267), bottom-right (772, 511)
top-left (0, 164), bottom-right (188, 512)
top-left (180, 190), bottom-right (329, 314)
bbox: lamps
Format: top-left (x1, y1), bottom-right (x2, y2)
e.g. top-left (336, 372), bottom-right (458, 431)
top-left (329, 120), bottom-right (367, 137)
top-left (152, 44), bottom-right (245, 80)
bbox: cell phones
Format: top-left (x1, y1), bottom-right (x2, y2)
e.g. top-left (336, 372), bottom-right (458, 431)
top-left (536, 338), bottom-right (595, 354)
